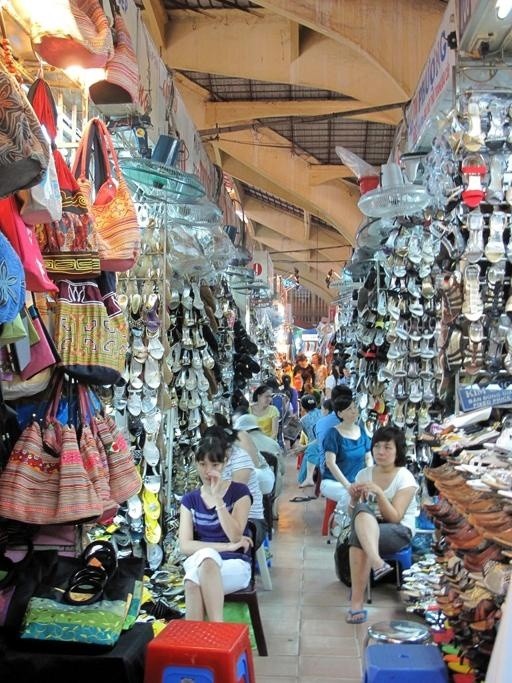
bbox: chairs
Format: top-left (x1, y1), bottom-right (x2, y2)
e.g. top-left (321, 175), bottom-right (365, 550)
top-left (224, 520), bottom-right (269, 655)
top-left (259, 450), bottom-right (279, 540)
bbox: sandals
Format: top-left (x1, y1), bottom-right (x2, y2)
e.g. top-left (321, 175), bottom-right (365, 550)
top-left (108, 286), bottom-right (261, 621)
top-left (69, 541), bottom-right (117, 605)
top-left (355, 89), bottom-right (511, 472)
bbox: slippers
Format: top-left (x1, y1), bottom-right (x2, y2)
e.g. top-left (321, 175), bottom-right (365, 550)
top-left (399, 427), bottom-right (512, 683)
top-left (370, 560), bottom-right (394, 582)
top-left (344, 601), bottom-right (369, 625)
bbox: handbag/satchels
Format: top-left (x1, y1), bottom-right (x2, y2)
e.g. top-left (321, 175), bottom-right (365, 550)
top-left (0, 0), bottom-right (144, 528)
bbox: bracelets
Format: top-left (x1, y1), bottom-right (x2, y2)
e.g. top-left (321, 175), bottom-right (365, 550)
top-left (214, 503), bottom-right (226, 510)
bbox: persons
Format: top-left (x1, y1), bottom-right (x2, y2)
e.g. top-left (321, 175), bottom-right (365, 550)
top-left (345, 425), bottom-right (419, 624)
top-left (232, 353), bottom-right (373, 553)
top-left (178, 435), bottom-right (254, 623)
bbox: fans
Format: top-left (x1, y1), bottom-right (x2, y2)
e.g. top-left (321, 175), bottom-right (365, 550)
top-left (354, 216), bottom-right (401, 253)
top-left (112, 112), bottom-right (207, 205)
top-left (357, 149), bottom-right (433, 218)
top-left (152, 196), bottom-right (224, 230)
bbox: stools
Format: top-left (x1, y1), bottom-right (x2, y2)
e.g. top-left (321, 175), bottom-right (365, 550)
top-left (362, 619), bottom-right (435, 672)
top-left (141, 617), bottom-right (257, 681)
top-left (321, 497), bottom-right (338, 536)
top-left (360, 642), bottom-right (450, 681)
top-left (256, 539), bottom-right (276, 590)
top-left (347, 544), bottom-right (414, 601)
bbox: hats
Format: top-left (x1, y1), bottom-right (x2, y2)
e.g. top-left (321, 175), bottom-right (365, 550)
top-left (294, 353), bottom-right (307, 363)
